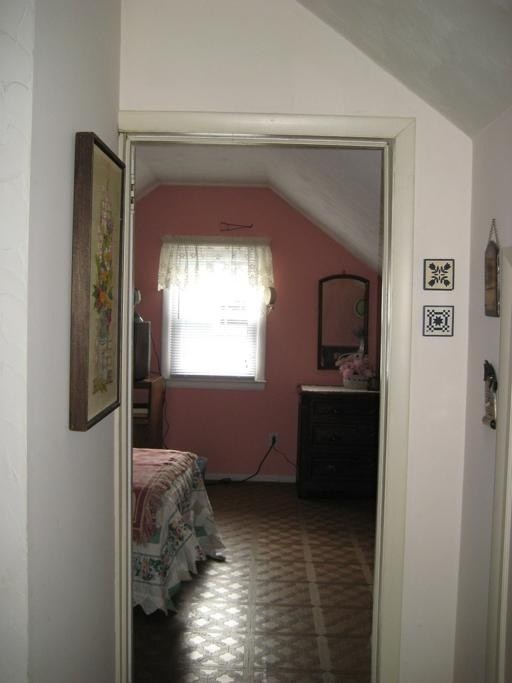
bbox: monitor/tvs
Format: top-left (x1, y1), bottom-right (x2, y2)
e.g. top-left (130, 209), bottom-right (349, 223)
top-left (133, 320), bottom-right (150, 380)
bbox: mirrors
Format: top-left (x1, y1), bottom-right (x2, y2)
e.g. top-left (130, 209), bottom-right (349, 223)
top-left (318, 273), bottom-right (368, 370)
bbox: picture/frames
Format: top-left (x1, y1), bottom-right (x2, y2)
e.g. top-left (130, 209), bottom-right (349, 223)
top-left (68, 131), bottom-right (125, 432)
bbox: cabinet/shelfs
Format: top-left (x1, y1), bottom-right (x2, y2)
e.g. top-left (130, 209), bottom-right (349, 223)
top-left (295, 384), bottom-right (379, 500)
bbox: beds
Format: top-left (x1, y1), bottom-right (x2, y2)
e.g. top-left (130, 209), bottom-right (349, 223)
top-left (129, 448), bottom-right (225, 618)
